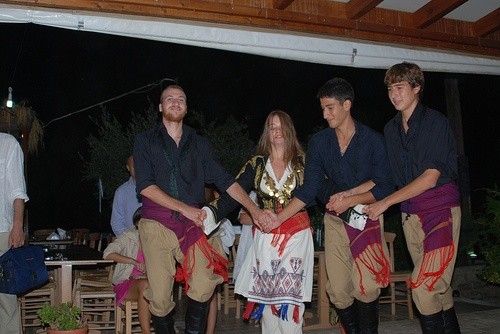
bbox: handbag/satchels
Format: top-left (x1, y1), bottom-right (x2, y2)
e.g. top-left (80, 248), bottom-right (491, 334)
top-left (0, 243), bottom-right (49, 298)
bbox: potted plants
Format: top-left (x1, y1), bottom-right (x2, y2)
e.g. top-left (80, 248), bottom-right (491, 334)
top-left (36, 300), bottom-right (88, 334)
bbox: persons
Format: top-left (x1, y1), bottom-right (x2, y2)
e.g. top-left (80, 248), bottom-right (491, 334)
top-left (131, 84), bottom-right (267, 333)
top-left (260, 77), bottom-right (387, 333)
top-left (328, 60), bottom-right (462, 334)
top-left (104, 151), bottom-right (152, 333)
top-left (198, 111), bottom-right (314, 333)
top-left (0, 130), bottom-right (29, 333)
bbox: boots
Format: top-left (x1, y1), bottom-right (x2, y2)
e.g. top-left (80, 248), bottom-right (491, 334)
top-left (184, 295), bottom-right (211, 334)
top-left (412, 303), bottom-right (461, 334)
top-left (332, 297), bottom-right (358, 334)
top-left (356, 298), bottom-right (379, 334)
top-left (150, 307), bottom-right (176, 334)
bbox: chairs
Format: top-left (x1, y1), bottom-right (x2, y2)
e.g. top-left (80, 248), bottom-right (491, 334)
top-left (19, 228), bottom-right (415, 334)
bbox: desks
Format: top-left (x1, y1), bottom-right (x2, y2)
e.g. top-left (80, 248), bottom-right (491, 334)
top-left (28, 238), bottom-right (77, 245)
top-left (36, 245), bottom-right (114, 334)
top-left (302, 247), bottom-right (340, 330)
